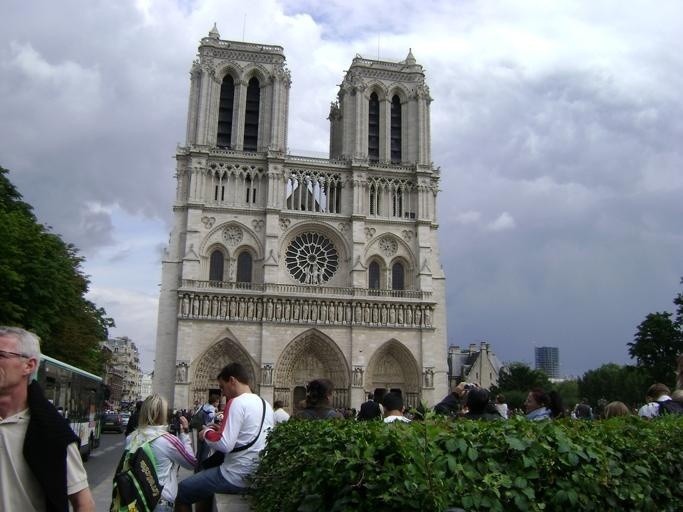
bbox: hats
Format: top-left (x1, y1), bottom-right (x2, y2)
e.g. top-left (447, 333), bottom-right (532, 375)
top-left (357, 400), bottom-right (381, 418)
top-left (464, 387), bottom-right (490, 407)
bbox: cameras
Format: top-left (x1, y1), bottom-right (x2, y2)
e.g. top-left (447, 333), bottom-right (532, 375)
top-left (171, 409), bottom-right (194, 431)
top-left (464, 384), bottom-right (475, 390)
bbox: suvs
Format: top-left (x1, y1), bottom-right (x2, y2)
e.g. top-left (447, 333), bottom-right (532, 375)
top-left (100, 413), bottom-right (123, 433)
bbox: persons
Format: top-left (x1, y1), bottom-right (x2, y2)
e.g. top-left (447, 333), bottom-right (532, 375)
top-left (434, 382), bottom-right (681, 421)
top-left (125, 392), bottom-right (198, 512)
top-left (126, 394), bottom-right (224, 461)
top-left (175, 361), bottom-right (274, 511)
top-left (273, 379), bottom-right (412, 425)
top-left (0, 325), bottom-right (96, 511)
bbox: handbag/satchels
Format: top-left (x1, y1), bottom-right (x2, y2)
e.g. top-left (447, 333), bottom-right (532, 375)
top-left (201, 450), bottom-right (224, 469)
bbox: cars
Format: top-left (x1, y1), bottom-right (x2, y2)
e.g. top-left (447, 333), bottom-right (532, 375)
top-left (119, 413), bottom-right (130, 426)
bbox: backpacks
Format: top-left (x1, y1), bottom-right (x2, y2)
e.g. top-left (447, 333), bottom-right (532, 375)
top-left (110, 428), bottom-right (175, 512)
top-left (190, 404), bottom-right (213, 428)
top-left (652, 399), bottom-right (683, 416)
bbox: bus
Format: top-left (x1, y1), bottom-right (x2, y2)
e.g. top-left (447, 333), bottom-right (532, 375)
top-left (26, 353), bottom-right (111, 464)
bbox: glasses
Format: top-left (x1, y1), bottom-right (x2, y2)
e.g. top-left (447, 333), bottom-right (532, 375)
top-left (1, 350), bottom-right (29, 358)
